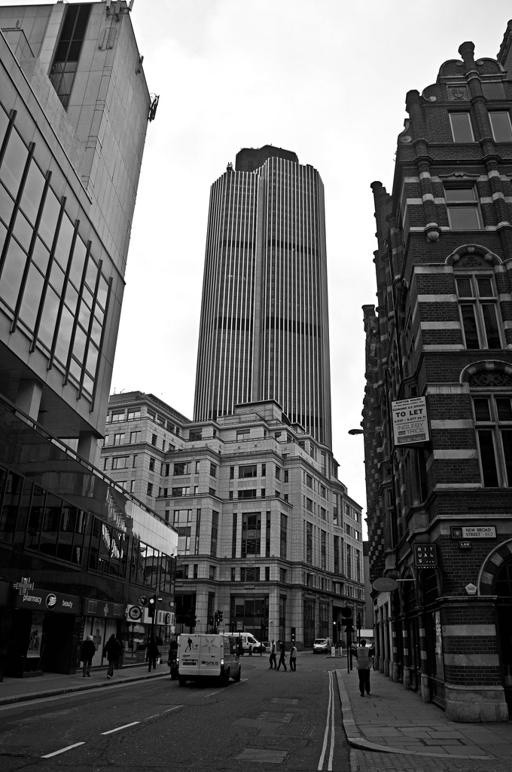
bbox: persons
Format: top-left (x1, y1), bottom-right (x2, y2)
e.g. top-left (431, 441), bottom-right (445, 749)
top-left (145, 637), bottom-right (162, 673)
top-left (353, 640), bottom-right (373, 697)
top-left (277, 640), bottom-right (287, 671)
top-left (268, 640), bottom-right (276, 670)
top-left (102, 633), bottom-right (122, 680)
top-left (289, 642), bottom-right (297, 671)
top-left (79, 634), bottom-right (96, 678)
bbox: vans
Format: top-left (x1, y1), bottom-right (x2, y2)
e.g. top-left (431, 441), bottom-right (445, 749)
top-left (128, 624), bottom-right (145, 648)
top-left (177, 632), bottom-right (266, 687)
top-left (354, 629), bottom-right (375, 656)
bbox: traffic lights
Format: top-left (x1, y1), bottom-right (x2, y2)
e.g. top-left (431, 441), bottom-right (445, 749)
top-left (342, 606), bottom-right (356, 632)
top-left (148, 596), bottom-right (156, 617)
top-left (291, 627), bottom-right (296, 642)
top-left (208, 610), bottom-right (223, 626)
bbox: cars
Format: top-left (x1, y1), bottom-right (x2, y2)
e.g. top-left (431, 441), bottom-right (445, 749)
top-left (313, 638), bottom-right (333, 654)
